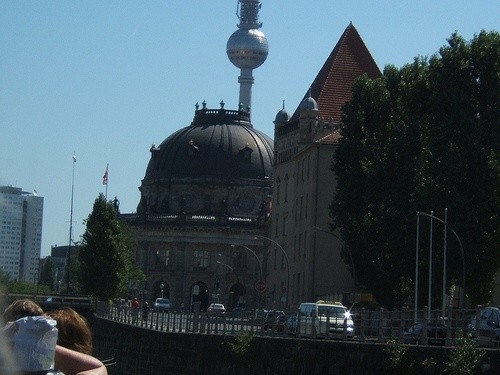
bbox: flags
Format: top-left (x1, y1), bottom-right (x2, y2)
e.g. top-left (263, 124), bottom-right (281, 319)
top-left (103, 168), bottom-right (108, 184)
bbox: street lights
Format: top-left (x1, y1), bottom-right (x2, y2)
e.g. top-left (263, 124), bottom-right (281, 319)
top-left (253, 234), bottom-right (290, 310)
top-left (417, 210), bottom-right (466, 327)
top-left (216, 252), bottom-right (245, 309)
top-left (309, 225), bottom-right (356, 313)
top-left (229, 242), bottom-right (264, 310)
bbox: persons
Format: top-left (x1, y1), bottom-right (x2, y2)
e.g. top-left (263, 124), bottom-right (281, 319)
top-left (115, 297), bottom-right (150, 323)
top-left (0, 299), bottom-right (108, 375)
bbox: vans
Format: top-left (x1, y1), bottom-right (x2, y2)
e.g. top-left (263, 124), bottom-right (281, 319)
top-left (296, 301), bottom-right (355, 341)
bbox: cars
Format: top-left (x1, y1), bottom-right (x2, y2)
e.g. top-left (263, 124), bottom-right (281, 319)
top-left (466, 305), bottom-right (500, 349)
top-left (154, 297), bottom-right (170, 313)
top-left (207, 303), bottom-right (227, 318)
top-left (398, 323), bottom-right (447, 345)
top-left (261, 310), bottom-right (297, 336)
